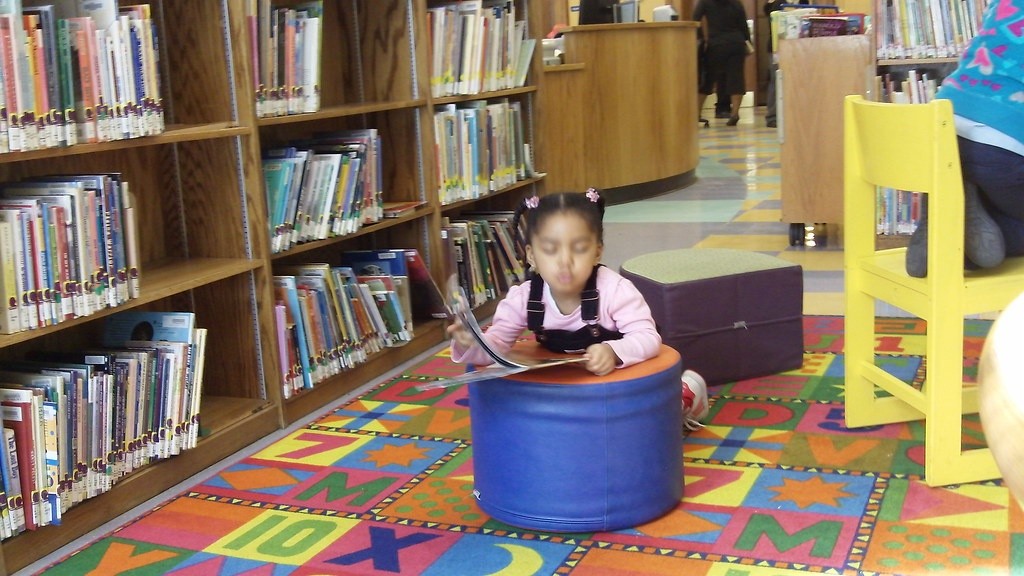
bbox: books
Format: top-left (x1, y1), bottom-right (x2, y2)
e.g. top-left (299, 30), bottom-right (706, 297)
top-left (421, 0), bottom-right (537, 314)
top-left (244, 0), bottom-right (324, 118)
top-left (259, 125), bottom-right (428, 254)
top-left (770, 0), bottom-right (1000, 60)
top-left (875, 66), bottom-right (944, 105)
top-left (269, 248), bottom-right (452, 400)
top-left (742, 41), bottom-right (755, 56)
top-left (0, 172), bottom-right (143, 340)
top-left (1, 306), bottom-right (212, 546)
top-left (0, 0), bottom-right (165, 154)
top-left (415, 284), bottom-right (591, 392)
top-left (876, 184), bottom-right (924, 236)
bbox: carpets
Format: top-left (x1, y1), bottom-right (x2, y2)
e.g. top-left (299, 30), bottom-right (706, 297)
top-left (28, 312), bottom-right (1023, 575)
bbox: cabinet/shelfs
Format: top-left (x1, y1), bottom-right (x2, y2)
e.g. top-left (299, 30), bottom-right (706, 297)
top-left (424, 0), bottom-right (550, 341)
top-left (0, 1), bottom-right (284, 575)
top-left (872, 0), bottom-right (993, 254)
top-left (243, 0), bottom-right (450, 429)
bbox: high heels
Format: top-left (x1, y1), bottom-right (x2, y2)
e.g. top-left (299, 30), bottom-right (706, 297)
top-left (727, 114), bottom-right (740, 126)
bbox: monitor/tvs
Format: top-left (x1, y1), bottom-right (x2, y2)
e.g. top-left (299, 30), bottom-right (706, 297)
top-left (578, 0), bottom-right (639, 25)
top-left (653, 4), bottom-right (679, 21)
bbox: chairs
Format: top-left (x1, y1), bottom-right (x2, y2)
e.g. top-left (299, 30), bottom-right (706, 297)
top-left (842, 93), bottom-right (1024, 486)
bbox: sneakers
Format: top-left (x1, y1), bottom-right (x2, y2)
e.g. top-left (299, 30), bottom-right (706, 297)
top-left (681, 368), bottom-right (709, 432)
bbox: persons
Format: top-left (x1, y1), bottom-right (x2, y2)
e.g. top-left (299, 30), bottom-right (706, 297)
top-left (764, 0), bottom-right (811, 128)
top-left (905, 0), bottom-right (1024, 282)
top-left (692, 0), bottom-right (752, 125)
top-left (448, 188), bottom-right (709, 431)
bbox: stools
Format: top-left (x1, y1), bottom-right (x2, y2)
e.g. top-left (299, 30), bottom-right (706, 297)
top-left (616, 248), bottom-right (807, 389)
top-left (465, 340), bottom-right (686, 532)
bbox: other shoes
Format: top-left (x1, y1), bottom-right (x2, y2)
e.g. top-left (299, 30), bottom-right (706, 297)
top-left (961, 178), bottom-right (1009, 272)
top-left (905, 192), bottom-right (929, 278)
